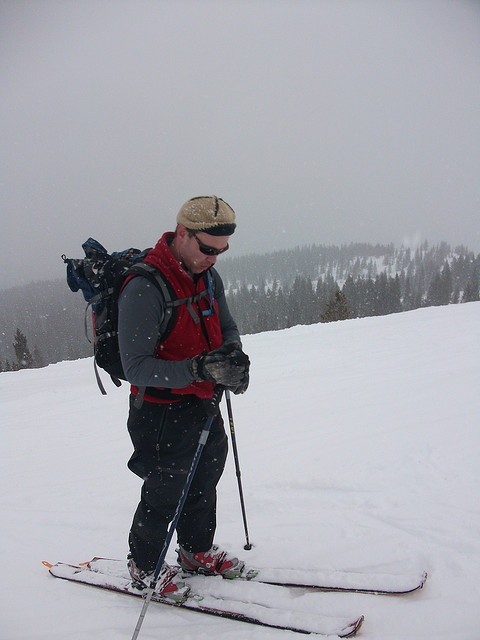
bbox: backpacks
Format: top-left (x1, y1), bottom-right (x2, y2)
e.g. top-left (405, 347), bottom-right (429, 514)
top-left (61, 237), bottom-right (214, 395)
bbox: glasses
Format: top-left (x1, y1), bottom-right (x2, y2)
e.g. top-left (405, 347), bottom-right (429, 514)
top-left (192, 231), bottom-right (229, 256)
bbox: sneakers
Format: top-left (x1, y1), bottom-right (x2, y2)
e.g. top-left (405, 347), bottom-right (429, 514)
top-left (126, 556), bottom-right (192, 604)
top-left (175, 541), bottom-right (242, 580)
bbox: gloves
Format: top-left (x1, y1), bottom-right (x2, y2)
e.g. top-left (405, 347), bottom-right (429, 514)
top-left (197, 340), bottom-right (250, 386)
top-left (230, 376), bottom-right (249, 395)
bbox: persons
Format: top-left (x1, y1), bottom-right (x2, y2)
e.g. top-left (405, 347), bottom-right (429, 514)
top-left (117, 195), bottom-right (246, 602)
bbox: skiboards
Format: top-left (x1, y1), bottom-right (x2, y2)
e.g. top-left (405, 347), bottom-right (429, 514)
top-left (42, 557), bottom-right (427, 638)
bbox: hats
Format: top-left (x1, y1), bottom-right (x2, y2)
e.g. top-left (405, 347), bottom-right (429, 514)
top-left (177, 194), bottom-right (237, 236)
